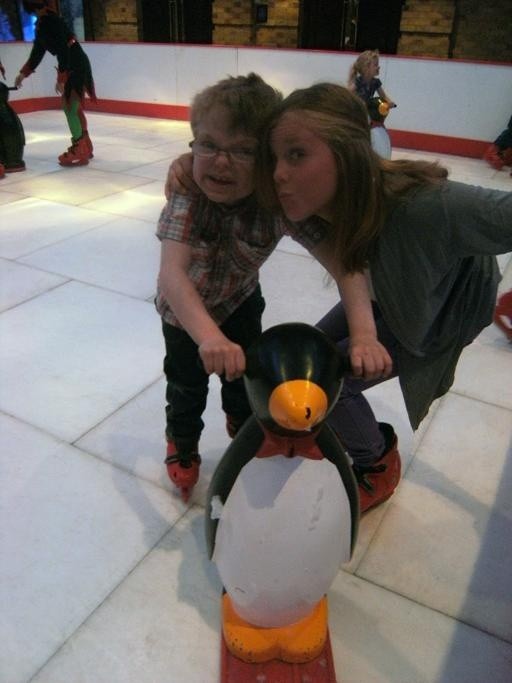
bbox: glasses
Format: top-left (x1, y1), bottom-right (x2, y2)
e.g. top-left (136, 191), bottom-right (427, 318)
top-left (189, 138), bottom-right (258, 167)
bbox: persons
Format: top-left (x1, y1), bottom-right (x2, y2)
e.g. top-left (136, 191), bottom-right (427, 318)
top-left (347, 48), bottom-right (394, 107)
top-left (12, 0), bottom-right (98, 163)
top-left (483, 116), bottom-right (511, 170)
top-left (150, 70), bottom-right (392, 493)
top-left (262, 81), bottom-right (511, 516)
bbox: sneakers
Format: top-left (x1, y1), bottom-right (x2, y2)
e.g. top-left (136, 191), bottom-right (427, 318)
top-left (225, 412), bottom-right (254, 436)
top-left (164, 416), bottom-right (204, 488)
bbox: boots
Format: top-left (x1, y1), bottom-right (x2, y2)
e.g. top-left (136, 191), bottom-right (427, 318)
top-left (350, 423), bottom-right (402, 519)
top-left (58, 130), bottom-right (94, 161)
